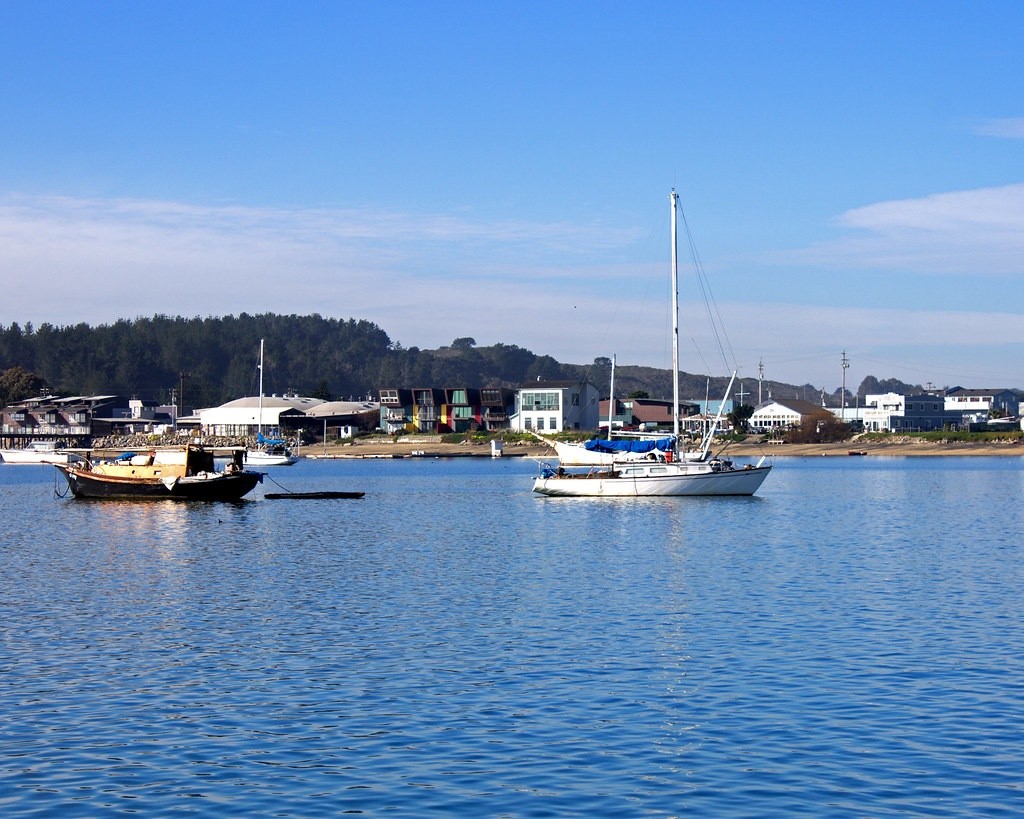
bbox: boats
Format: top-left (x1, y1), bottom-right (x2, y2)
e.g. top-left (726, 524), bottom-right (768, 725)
top-left (532, 371), bottom-right (774, 496)
top-left (849, 451), bottom-right (867, 455)
top-left (42, 442), bottom-right (269, 501)
top-left (265, 492), bottom-right (366, 499)
top-left (0, 441), bottom-right (82, 463)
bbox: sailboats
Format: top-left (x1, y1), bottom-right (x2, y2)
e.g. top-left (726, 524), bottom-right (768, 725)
top-left (531, 187), bottom-right (744, 466)
top-left (191, 339), bottom-right (301, 466)
